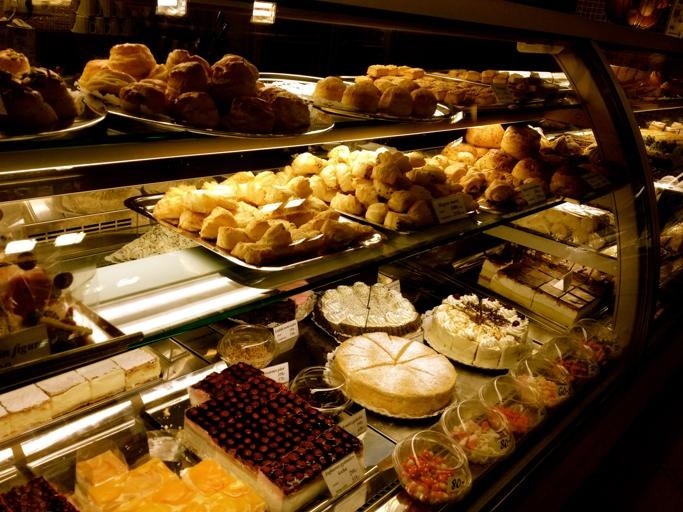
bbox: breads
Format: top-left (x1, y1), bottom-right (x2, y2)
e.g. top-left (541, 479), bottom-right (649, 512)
top-left (318, 65), bottom-right (436, 116)
top-left (77, 42), bottom-right (311, 132)
top-left (284, 147), bottom-right (478, 230)
top-left (0, 49), bottom-right (76, 136)
top-left (372, 60), bottom-right (564, 106)
top-left (152, 171), bottom-right (373, 265)
top-left (429, 123), bottom-right (611, 206)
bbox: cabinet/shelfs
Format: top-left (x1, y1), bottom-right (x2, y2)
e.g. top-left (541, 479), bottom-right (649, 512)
top-left (0, 0), bottom-right (682, 510)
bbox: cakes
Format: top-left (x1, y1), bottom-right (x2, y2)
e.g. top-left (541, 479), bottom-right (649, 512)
top-left (0, 251), bottom-right (93, 349)
top-left (0, 477), bottom-right (73, 512)
top-left (327, 331), bottom-right (456, 415)
top-left (640, 121), bottom-right (683, 171)
top-left (517, 201), bottom-right (608, 246)
top-left (417, 293), bottom-right (528, 369)
top-left (310, 279), bottom-right (420, 338)
top-left (70, 439), bottom-right (268, 512)
top-left (0, 346), bottom-right (162, 431)
top-left (179, 361), bottom-right (363, 512)
top-left (217, 324), bottom-right (275, 367)
top-left (220, 281), bottom-right (314, 323)
top-left (478, 248), bottom-right (603, 327)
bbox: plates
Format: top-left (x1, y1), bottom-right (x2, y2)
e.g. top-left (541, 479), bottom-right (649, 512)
top-left (99, 97), bottom-right (337, 140)
top-left (0, 104), bottom-right (106, 147)
top-left (255, 71), bottom-right (463, 126)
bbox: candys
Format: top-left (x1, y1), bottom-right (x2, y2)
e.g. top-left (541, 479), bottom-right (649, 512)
top-left (494, 402), bottom-right (530, 430)
top-left (449, 417), bottom-right (504, 458)
top-left (394, 428), bottom-right (458, 500)
top-left (521, 373), bottom-right (562, 409)
